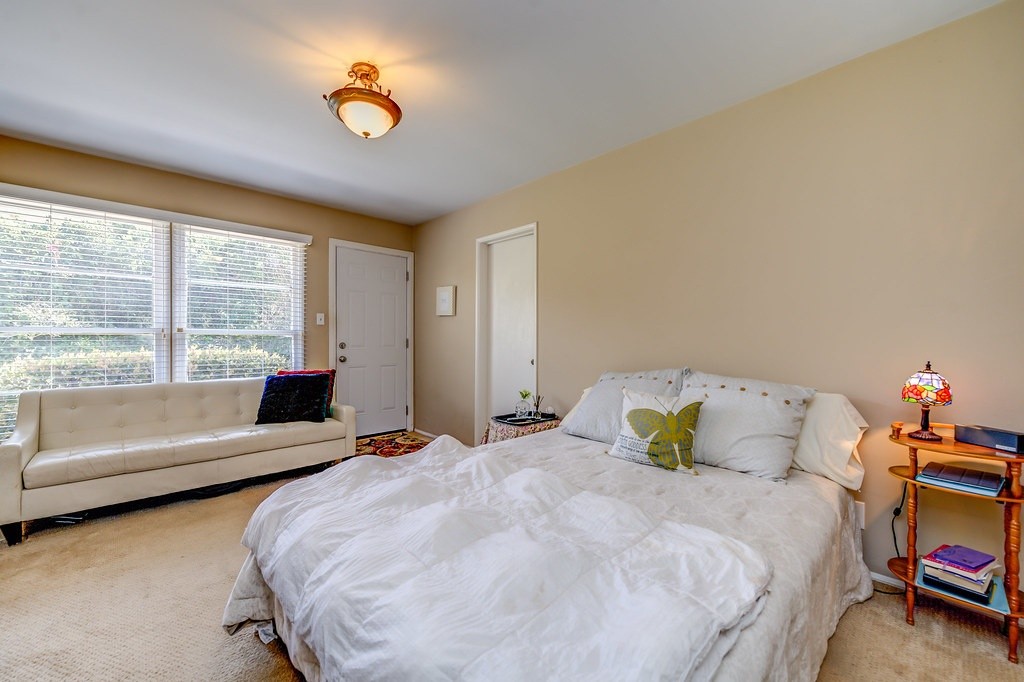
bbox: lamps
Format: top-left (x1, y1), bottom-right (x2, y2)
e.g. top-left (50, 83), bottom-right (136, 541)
top-left (901, 360), bottom-right (955, 442)
top-left (322, 61), bottom-right (402, 139)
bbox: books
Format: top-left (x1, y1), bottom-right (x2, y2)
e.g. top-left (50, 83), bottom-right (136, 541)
top-left (914, 543), bottom-right (1011, 615)
top-left (915, 462), bottom-right (1005, 497)
top-left (51, 512), bottom-right (89, 524)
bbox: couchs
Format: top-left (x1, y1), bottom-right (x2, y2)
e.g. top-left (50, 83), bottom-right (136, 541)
top-left (0, 376), bottom-right (357, 540)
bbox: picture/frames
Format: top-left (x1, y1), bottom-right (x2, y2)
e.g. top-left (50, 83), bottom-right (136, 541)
top-left (436, 285), bottom-right (456, 316)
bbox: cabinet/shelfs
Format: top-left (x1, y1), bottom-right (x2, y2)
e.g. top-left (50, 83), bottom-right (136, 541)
top-left (887, 433), bottom-right (1024, 664)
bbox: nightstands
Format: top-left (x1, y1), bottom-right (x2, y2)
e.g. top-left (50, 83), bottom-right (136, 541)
top-left (489, 412), bottom-right (561, 442)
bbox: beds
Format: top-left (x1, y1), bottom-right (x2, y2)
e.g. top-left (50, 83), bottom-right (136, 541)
top-left (219, 385), bottom-right (873, 682)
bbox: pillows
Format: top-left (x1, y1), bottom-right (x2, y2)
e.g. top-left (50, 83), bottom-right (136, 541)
top-left (255, 373), bottom-right (329, 425)
top-left (605, 386), bottom-right (705, 476)
top-left (673, 367), bottom-right (818, 487)
top-left (277, 369), bottom-right (337, 418)
top-left (560, 368), bottom-right (692, 446)
